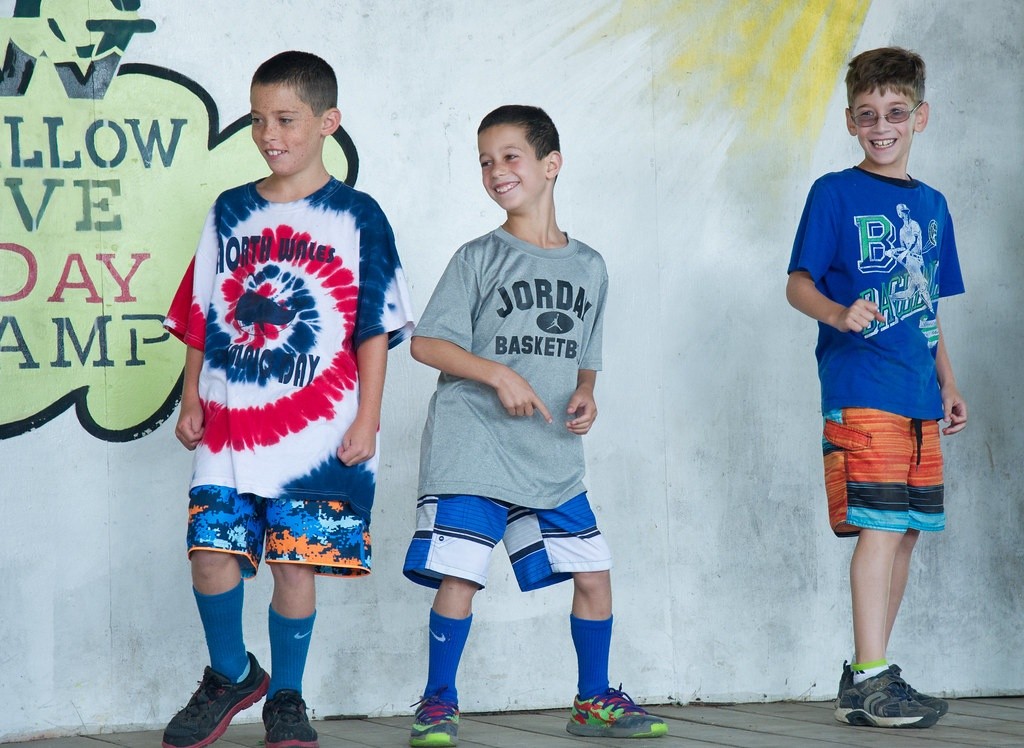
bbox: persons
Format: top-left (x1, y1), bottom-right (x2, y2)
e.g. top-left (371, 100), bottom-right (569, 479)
top-left (159, 49), bottom-right (418, 747)
top-left (401, 104), bottom-right (670, 747)
top-left (786, 46), bottom-right (967, 728)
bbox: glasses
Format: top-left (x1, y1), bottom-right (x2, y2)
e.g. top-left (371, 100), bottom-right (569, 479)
top-left (849, 101), bottom-right (924, 127)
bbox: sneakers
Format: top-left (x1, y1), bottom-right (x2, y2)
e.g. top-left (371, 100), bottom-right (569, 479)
top-left (262, 689), bottom-right (319, 748)
top-left (567, 694), bottom-right (668, 737)
top-left (162, 650), bottom-right (271, 748)
top-left (834, 664), bottom-right (949, 717)
top-left (834, 672), bottom-right (938, 729)
top-left (409, 698), bottom-right (460, 746)
